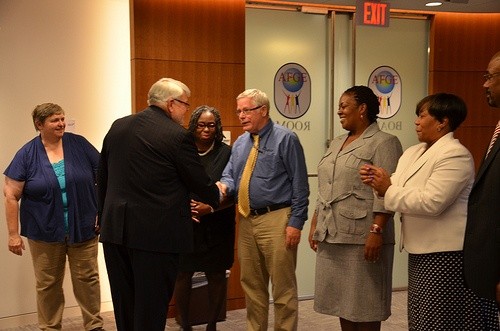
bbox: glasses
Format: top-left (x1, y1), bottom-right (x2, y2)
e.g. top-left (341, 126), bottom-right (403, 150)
top-left (167, 97), bottom-right (191, 108)
top-left (483, 71), bottom-right (500, 81)
top-left (196, 121), bottom-right (216, 129)
top-left (235, 104), bottom-right (266, 115)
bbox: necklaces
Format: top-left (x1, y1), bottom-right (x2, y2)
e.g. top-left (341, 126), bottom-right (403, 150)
top-left (198, 140), bottom-right (215, 156)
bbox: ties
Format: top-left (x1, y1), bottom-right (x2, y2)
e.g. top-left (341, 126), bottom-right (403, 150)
top-left (485, 120), bottom-right (500, 160)
top-left (238, 135), bottom-right (259, 218)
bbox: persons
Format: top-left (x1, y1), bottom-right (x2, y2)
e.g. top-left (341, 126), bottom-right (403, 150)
top-left (461, 51), bottom-right (500, 313)
top-left (309, 86), bottom-right (404, 331)
top-left (220, 89), bottom-right (311, 331)
top-left (360, 93), bottom-right (500, 331)
top-left (3, 103), bottom-right (106, 331)
top-left (174, 105), bottom-right (237, 331)
top-left (97, 78), bottom-right (225, 331)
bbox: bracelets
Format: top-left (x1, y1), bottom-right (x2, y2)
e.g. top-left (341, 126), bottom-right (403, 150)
top-left (370, 223), bottom-right (384, 235)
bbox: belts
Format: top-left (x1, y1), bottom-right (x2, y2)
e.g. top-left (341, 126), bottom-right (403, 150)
top-left (250, 200), bottom-right (291, 215)
